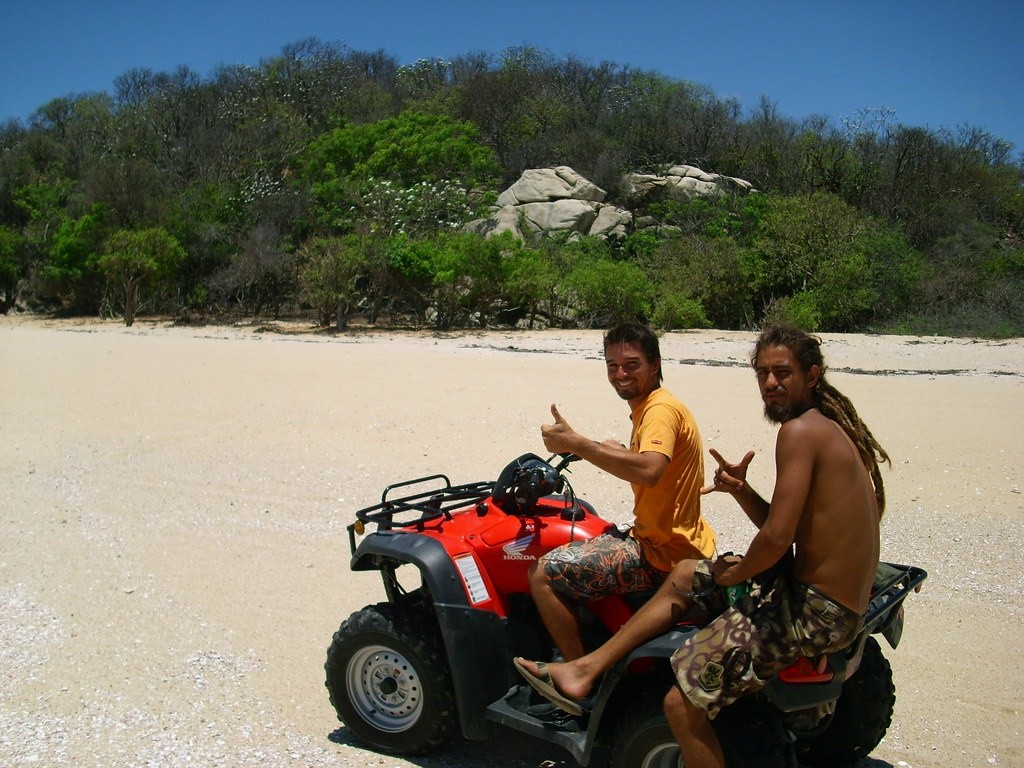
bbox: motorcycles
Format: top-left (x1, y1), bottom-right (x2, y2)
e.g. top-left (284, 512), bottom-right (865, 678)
top-left (323, 443), bottom-right (928, 767)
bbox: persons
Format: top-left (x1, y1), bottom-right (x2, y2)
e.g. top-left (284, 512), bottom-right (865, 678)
top-left (512, 325), bottom-right (893, 767)
top-left (526, 324), bottom-right (719, 718)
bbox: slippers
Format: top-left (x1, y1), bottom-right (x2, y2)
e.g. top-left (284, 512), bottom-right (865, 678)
top-left (512, 656), bottom-right (584, 718)
